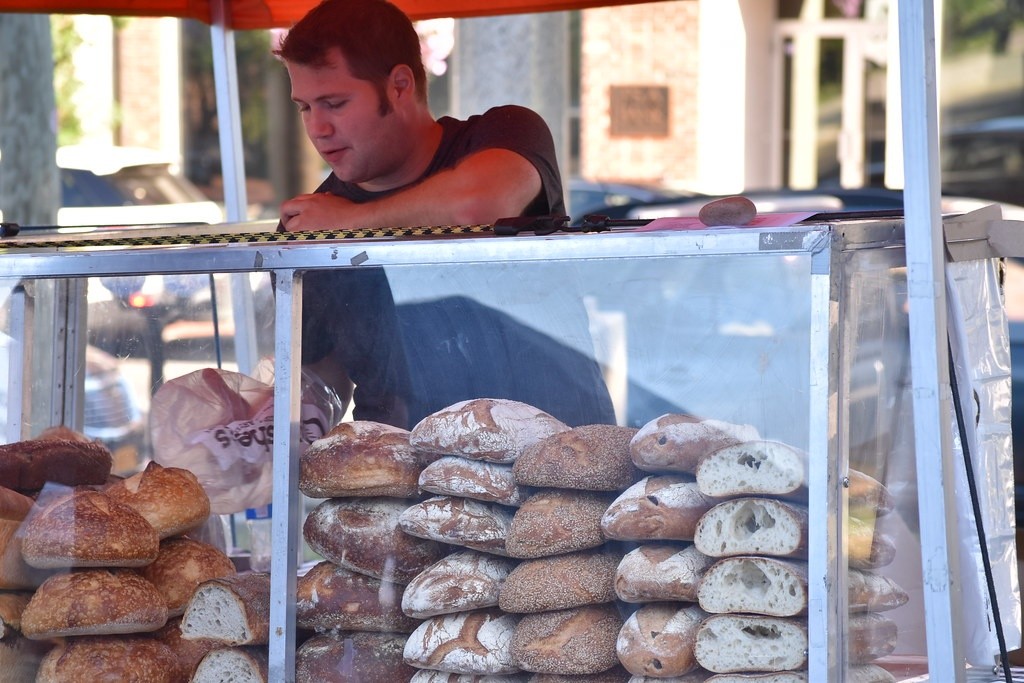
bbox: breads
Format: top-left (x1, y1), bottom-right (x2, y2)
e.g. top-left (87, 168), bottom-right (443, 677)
top-left (0, 425), bottom-right (273, 683)
top-left (294, 396), bottom-right (910, 683)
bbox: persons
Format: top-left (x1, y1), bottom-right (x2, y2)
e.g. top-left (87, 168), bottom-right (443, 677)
top-left (187, 0), bottom-right (617, 490)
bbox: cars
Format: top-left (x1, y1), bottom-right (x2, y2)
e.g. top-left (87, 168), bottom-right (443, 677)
top-left (569, 179), bottom-right (1024, 318)
top-left (0, 331), bottom-right (142, 462)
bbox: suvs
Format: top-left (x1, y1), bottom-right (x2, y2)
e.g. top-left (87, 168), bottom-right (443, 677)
top-left (56, 140), bottom-right (227, 331)
